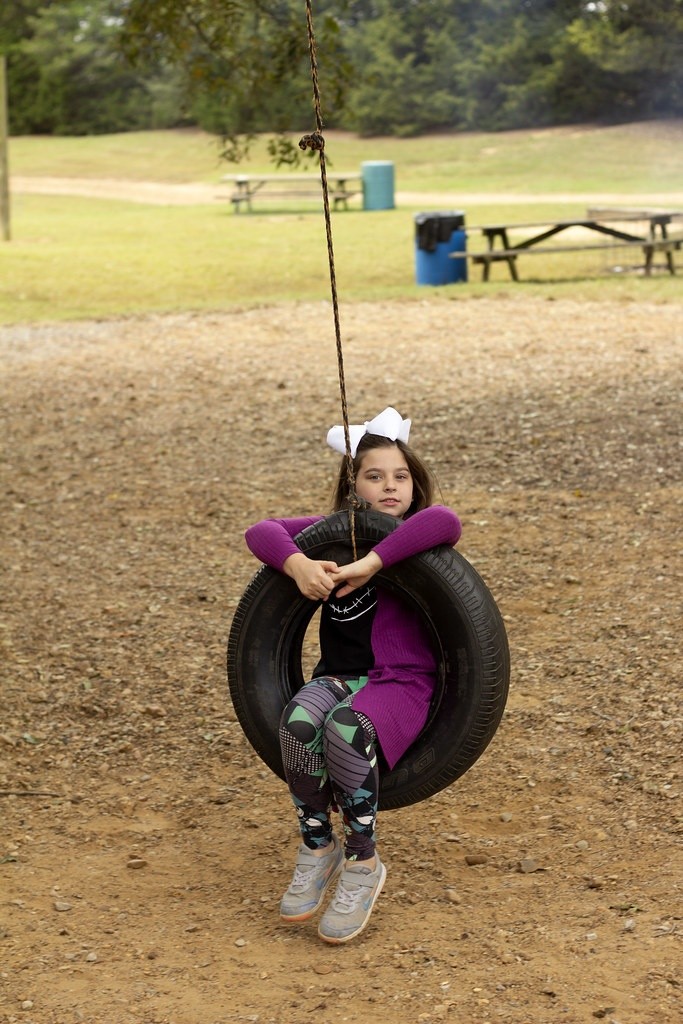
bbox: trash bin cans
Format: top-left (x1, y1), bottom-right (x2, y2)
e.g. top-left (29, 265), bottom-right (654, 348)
top-left (414, 209), bottom-right (470, 284)
top-left (362, 161), bottom-right (394, 211)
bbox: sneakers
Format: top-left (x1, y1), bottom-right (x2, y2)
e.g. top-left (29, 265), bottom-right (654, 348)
top-left (280, 833), bottom-right (345, 922)
top-left (318, 847), bottom-right (386, 944)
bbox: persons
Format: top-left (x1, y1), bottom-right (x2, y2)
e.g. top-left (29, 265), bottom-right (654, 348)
top-left (245, 408), bottom-right (462, 943)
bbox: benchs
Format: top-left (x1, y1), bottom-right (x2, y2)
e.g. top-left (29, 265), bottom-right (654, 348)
top-left (448, 237), bottom-right (682, 264)
top-left (230, 189), bottom-right (361, 203)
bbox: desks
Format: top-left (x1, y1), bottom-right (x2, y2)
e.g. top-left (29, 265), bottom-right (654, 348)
top-left (458, 207), bottom-right (683, 282)
top-left (221, 172), bottom-right (361, 215)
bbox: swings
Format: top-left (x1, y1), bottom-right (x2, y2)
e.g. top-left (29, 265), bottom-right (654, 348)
top-left (227, 3), bottom-right (506, 812)
